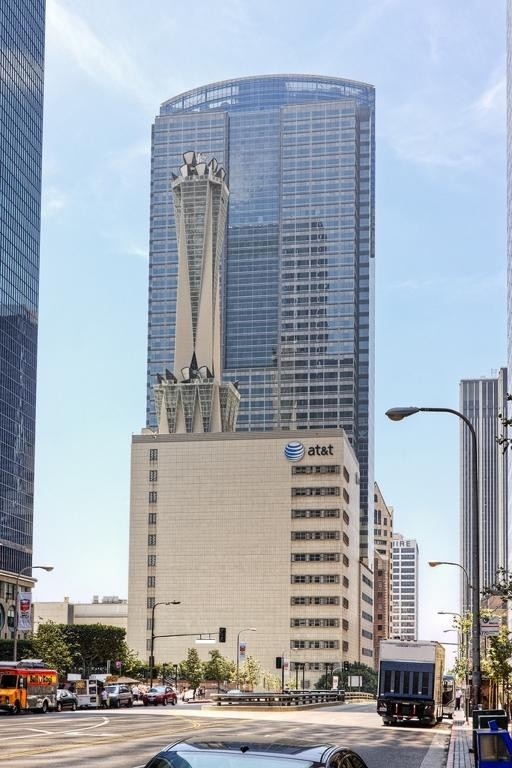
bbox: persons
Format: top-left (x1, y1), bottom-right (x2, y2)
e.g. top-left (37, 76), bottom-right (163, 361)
top-left (100, 686), bottom-right (109, 708)
top-left (131, 684), bottom-right (139, 701)
top-left (68, 682), bottom-right (75, 693)
top-left (455, 688), bottom-right (463, 710)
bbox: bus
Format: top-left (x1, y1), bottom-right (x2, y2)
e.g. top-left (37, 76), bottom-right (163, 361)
top-left (442, 675), bottom-right (456, 719)
top-left (0, 659), bottom-right (58, 715)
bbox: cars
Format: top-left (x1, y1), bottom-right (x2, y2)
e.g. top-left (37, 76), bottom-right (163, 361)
top-left (57, 689), bottom-right (79, 711)
top-left (142, 685), bottom-right (178, 706)
top-left (226, 690), bottom-right (242, 695)
top-left (140, 736), bottom-right (366, 768)
top-left (104, 685), bottom-right (133, 708)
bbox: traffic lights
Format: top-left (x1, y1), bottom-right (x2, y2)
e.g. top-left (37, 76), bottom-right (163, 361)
top-left (343, 661), bottom-right (349, 671)
top-left (218, 627), bottom-right (226, 643)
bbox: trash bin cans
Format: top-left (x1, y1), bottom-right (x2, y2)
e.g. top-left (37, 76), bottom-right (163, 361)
top-left (455, 698), bottom-right (460, 710)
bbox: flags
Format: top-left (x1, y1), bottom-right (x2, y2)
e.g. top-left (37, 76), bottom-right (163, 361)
top-left (284, 657), bottom-right (291, 671)
top-left (332, 675), bottom-right (339, 689)
top-left (239, 641), bottom-right (248, 662)
top-left (16, 591), bottom-right (32, 630)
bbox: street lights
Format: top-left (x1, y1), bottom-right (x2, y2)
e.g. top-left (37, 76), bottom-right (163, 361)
top-left (150, 600), bottom-right (181, 689)
top-left (427, 561), bottom-right (469, 671)
top-left (12, 565), bottom-right (55, 661)
top-left (384, 406), bottom-right (480, 714)
top-left (281, 648), bottom-right (298, 692)
top-left (236, 627), bottom-right (257, 690)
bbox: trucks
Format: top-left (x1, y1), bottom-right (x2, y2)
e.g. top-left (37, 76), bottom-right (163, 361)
top-left (376, 638), bottom-right (445, 727)
